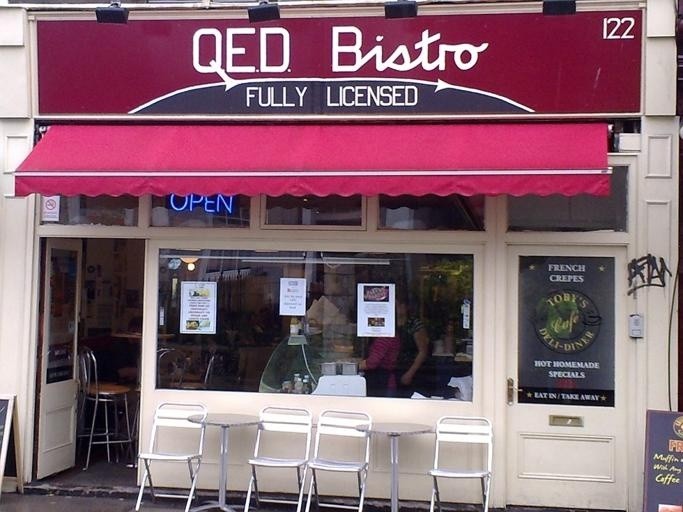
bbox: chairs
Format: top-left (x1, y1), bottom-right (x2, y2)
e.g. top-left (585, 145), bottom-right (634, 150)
top-left (244, 405), bottom-right (312, 511)
top-left (428, 416), bottom-right (494, 511)
top-left (76, 345), bottom-right (136, 472)
top-left (305, 409), bottom-right (372, 511)
top-left (169, 355), bottom-right (215, 390)
top-left (136, 402), bottom-right (208, 512)
top-left (125, 348), bottom-right (187, 467)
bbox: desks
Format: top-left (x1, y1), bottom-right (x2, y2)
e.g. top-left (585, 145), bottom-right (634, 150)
top-left (364, 369), bottom-right (386, 383)
top-left (357, 422), bottom-right (432, 512)
top-left (188, 413), bottom-right (263, 512)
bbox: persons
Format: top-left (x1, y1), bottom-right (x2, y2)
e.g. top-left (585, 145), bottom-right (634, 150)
top-left (359, 332), bottom-right (399, 398)
top-left (396, 295), bottom-right (430, 398)
